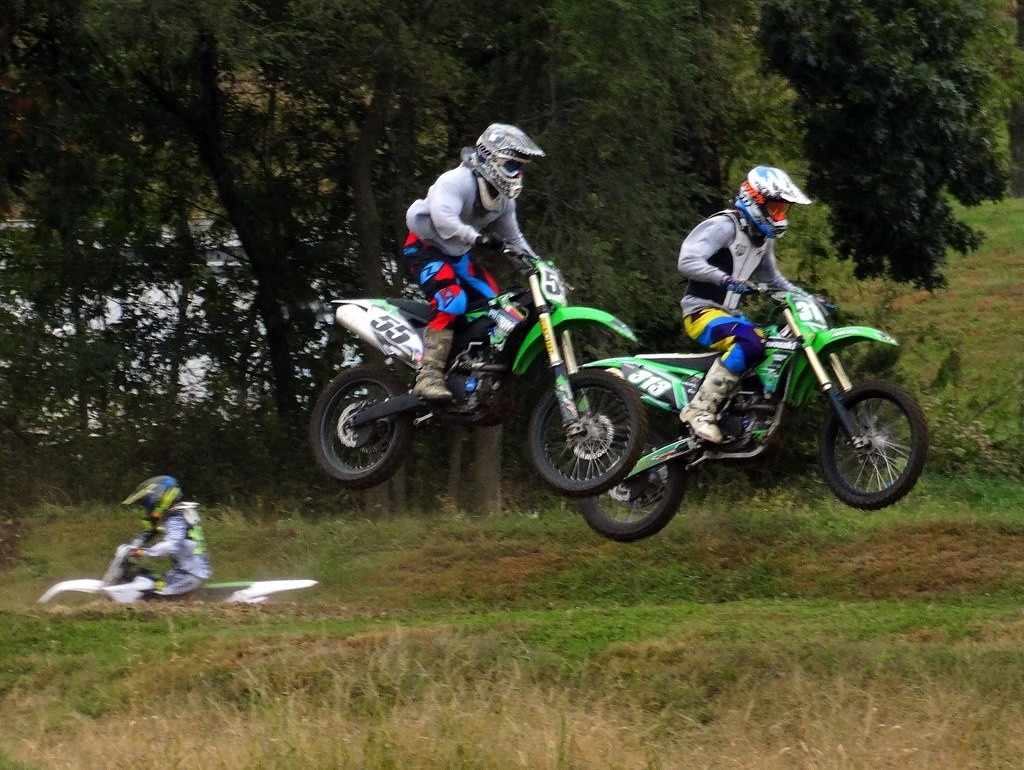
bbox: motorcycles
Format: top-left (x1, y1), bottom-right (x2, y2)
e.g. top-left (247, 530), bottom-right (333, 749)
top-left (573, 281), bottom-right (929, 540)
top-left (308, 233), bottom-right (649, 500)
top-left (38, 530), bottom-right (319, 604)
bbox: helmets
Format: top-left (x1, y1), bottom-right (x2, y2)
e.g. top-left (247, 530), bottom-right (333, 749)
top-left (471, 123), bottom-right (546, 202)
top-left (120, 474), bottom-right (182, 532)
top-left (733, 164), bottom-right (814, 239)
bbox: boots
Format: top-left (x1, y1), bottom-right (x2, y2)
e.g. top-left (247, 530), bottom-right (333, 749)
top-left (412, 326), bottom-right (454, 399)
top-left (680, 358), bottom-right (739, 443)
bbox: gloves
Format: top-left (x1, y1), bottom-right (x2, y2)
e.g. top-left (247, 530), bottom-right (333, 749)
top-left (723, 275), bottom-right (759, 295)
top-left (477, 233), bottom-right (535, 274)
top-left (810, 293), bottom-right (831, 310)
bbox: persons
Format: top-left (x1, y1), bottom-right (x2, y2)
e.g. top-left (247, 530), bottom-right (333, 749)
top-left (119, 475), bottom-right (212, 605)
top-left (399, 122), bottom-right (546, 401)
top-left (677, 165), bottom-right (820, 442)
